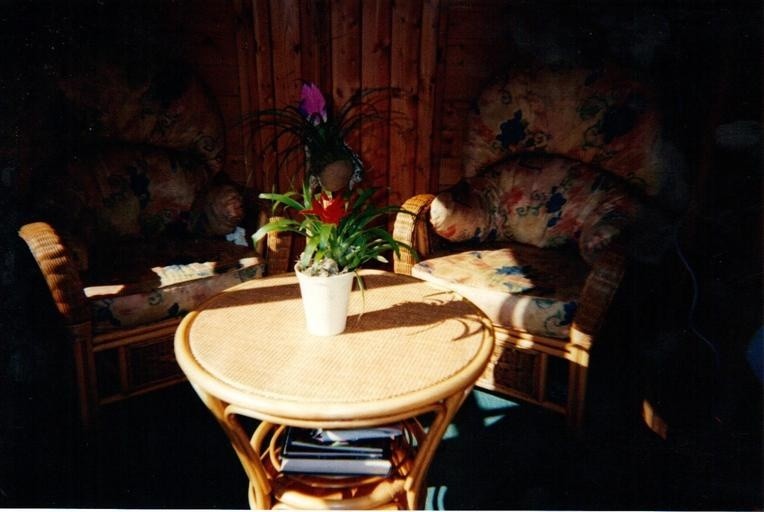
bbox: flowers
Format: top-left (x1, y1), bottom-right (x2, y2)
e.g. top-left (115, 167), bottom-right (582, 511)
top-left (234, 84), bottom-right (410, 185)
top-left (253, 181), bottom-right (424, 329)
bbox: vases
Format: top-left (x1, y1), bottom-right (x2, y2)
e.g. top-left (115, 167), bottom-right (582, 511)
top-left (318, 159), bottom-right (353, 194)
top-left (295, 263), bottom-right (352, 338)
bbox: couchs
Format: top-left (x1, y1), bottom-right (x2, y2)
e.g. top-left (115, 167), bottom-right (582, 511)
top-left (392, 143), bottom-right (640, 421)
top-left (14, 132), bottom-right (290, 418)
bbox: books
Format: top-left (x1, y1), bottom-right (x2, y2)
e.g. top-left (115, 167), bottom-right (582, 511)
top-left (276, 427), bottom-right (392, 477)
